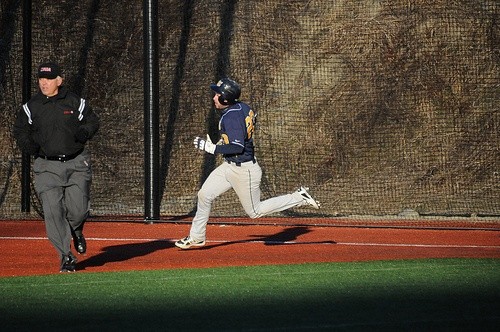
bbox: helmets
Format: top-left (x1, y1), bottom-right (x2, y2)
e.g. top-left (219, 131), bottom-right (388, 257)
top-left (209, 78), bottom-right (241, 105)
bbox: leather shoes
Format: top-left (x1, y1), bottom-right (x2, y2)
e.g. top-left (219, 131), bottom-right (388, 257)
top-left (72, 234), bottom-right (86, 253)
top-left (59, 256), bottom-right (76, 273)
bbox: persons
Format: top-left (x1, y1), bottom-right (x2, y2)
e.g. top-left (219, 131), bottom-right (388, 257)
top-left (12, 62), bottom-right (100, 272)
top-left (175, 79), bottom-right (321, 249)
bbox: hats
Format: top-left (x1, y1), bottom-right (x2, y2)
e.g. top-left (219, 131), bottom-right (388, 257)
top-left (35, 62), bottom-right (62, 78)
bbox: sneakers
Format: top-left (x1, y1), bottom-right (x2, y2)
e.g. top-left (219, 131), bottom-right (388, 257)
top-left (297, 185), bottom-right (321, 210)
top-left (175, 235), bottom-right (206, 249)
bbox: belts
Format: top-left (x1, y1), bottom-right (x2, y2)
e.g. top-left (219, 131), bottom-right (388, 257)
top-left (33, 148), bottom-right (84, 162)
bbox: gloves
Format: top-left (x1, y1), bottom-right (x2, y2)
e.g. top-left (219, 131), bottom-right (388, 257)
top-left (27, 142), bottom-right (39, 155)
top-left (194, 133), bottom-right (216, 154)
top-left (73, 129), bottom-right (88, 143)
